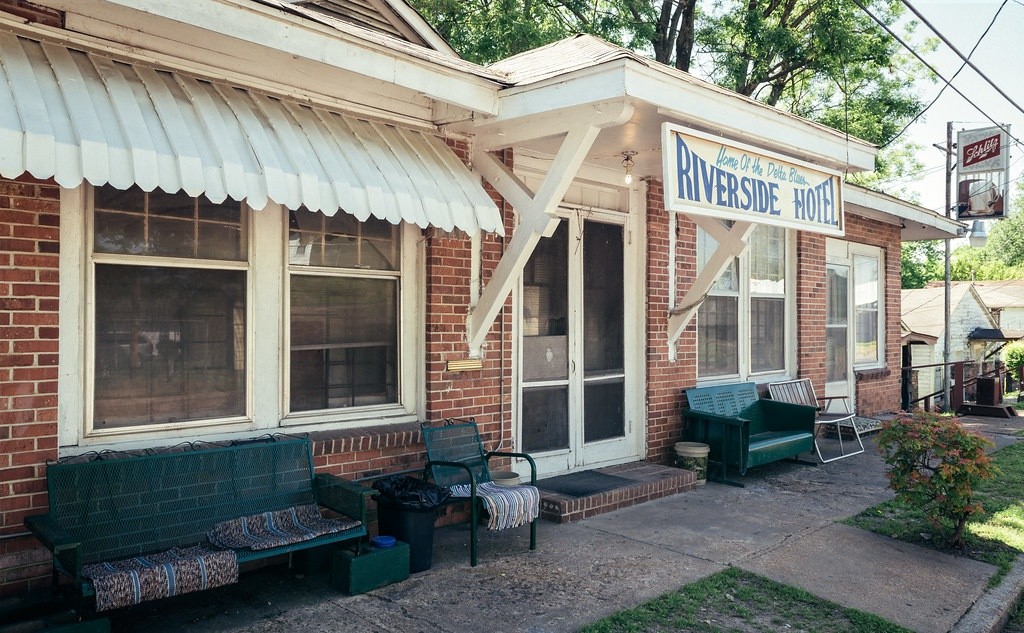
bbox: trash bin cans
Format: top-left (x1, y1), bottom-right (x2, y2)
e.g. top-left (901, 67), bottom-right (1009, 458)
top-left (370, 475), bottom-right (453, 572)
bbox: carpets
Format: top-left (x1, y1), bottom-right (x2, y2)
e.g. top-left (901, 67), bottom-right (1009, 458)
top-left (831, 417), bottom-right (884, 434)
top-left (521, 469), bottom-right (642, 499)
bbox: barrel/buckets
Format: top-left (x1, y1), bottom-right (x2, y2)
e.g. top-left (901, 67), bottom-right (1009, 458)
top-left (675, 441), bottom-right (710, 486)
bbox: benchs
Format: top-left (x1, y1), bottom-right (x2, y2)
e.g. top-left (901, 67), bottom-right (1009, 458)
top-left (24, 430), bottom-right (378, 620)
top-left (680, 382), bottom-right (822, 488)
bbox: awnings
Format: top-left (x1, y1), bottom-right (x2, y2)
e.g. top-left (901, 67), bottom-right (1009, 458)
top-left (0, 29), bottom-right (506, 238)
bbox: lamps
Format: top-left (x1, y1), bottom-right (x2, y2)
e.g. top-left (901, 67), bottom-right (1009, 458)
top-left (957, 219), bottom-right (988, 247)
top-left (621, 154), bottom-right (635, 184)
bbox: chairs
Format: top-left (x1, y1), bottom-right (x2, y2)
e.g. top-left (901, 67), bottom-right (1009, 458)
top-left (767, 377), bottom-right (865, 463)
top-left (420, 417), bottom-right (539, 566)
